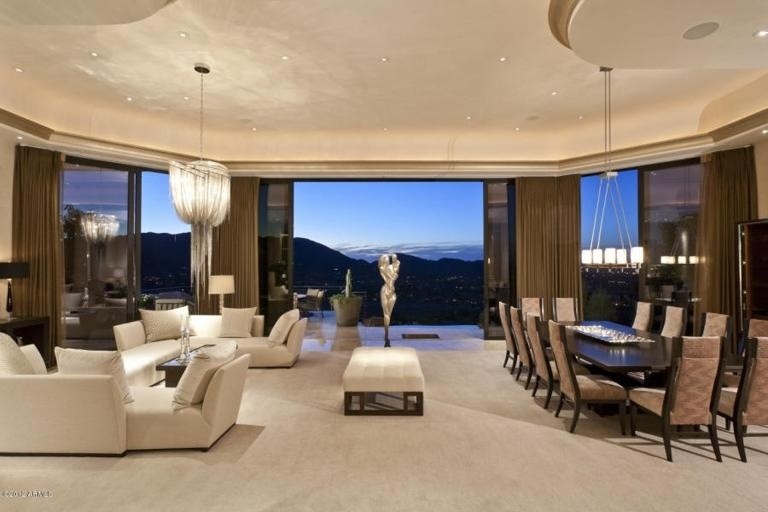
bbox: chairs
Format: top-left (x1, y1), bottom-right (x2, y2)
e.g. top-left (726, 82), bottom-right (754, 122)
top-left (499, 301), bottom-right (519, 374)
top-left (699, 312), bottom-right (733, 341)
top-left (298, 289), bottom-right (327, 318)
top-left (629, 336), bottom-right (727, 463)
top-left (509, 306), bottom-right (555, 390)
top-left (722, 319), bottom-right (768, 430)
top-left (552, 297), bottom-right (580, 322)
top-left (525, 312), bottom-right (591, 409)
top-left (297, 289), bottom-right (319, 300)
top-left (717, 337), bottom-right (768, 463)
top-left (548, 319), bottom-right (627, 435)
top-left (660, 305), bottom-right (687, 339)
top-left (520, 297), bottom-right (544, 322)
top-left (631, 301), bottom-right (654, 332)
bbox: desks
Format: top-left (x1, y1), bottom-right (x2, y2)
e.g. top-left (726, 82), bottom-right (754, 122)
top-left (0, 316), bottom-right (51, 368)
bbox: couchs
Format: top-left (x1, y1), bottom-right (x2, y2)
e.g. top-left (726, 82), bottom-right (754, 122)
top-left (0, 344), bottom-right (251, 455)
top-left (58, 307), bottom-right (128, 335)
top-left (342, 347), bottom-right (424, 416)
top-left (113, 314), bottom-right (308, 386)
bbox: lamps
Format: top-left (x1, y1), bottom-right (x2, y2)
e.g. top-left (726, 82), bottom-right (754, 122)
top-left (0, 261), bottom-right (30, 316)
top-left (80, 210), bottom-right (120, 264)
top-left (582, 67), bottom-right (644, 275)
top-left (169, 67), bottom-right (230, 307)
top-left (660, 166), bottom-right (706, 264)
top-left (208, 274), bottom-right (235, 312)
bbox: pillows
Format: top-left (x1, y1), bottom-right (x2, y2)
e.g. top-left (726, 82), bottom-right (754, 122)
top-left (216, 306), bottom-right (258, 337)
top-left (104, 297), bottom-right (136, 307)
top-left (268, 308), bottom-right (299, 349)
top-left (54, 346), bottom-right (135, 404)
top-left (171, 340), bottom-right (237, 410)
top-left (0, 332), bottom-right (37, 375)
top-left (137, 305), bottom-right (198, 343)
